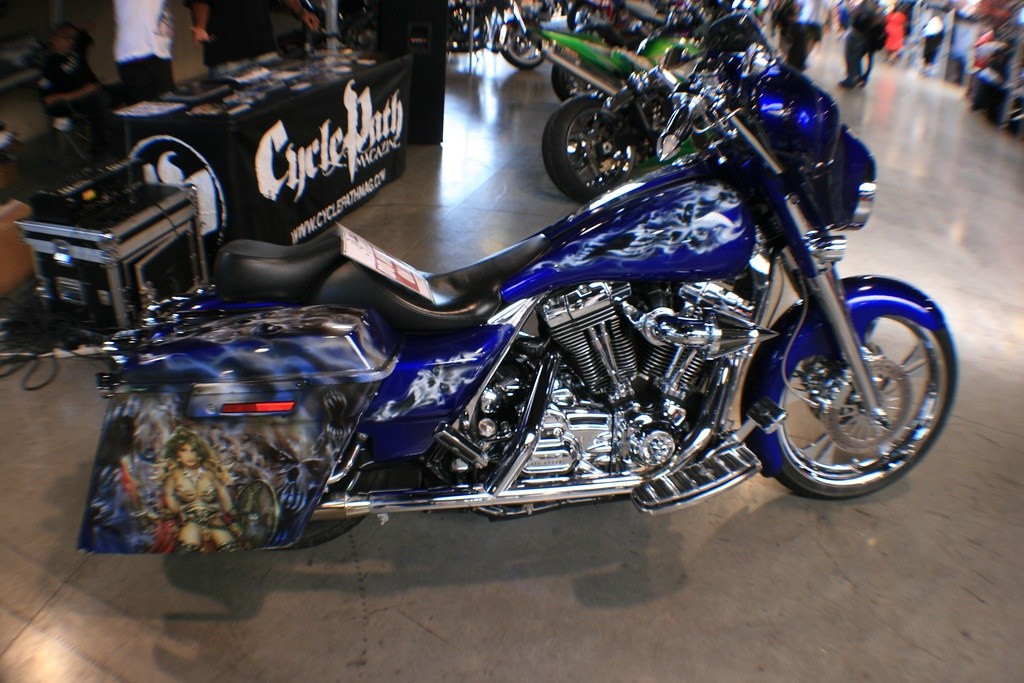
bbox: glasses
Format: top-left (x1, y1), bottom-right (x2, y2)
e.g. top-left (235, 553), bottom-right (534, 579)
top-left (58, 31), bottom-right (77, 41)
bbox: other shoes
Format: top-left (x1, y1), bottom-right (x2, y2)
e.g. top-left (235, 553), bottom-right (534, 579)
top-left (840, 74), bottom-right (861, 88)
top-left (86, 150), bottom-right (119, 164)
top-left (859, 74), bottom-right (867, 82)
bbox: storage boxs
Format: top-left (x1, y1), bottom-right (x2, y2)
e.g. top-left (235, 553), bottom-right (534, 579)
top-left (13, 181), bottom-right (208, 333)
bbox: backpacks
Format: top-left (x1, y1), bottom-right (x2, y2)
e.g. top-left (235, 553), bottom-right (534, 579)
top-left (866, 20), bottom-right (888, 54)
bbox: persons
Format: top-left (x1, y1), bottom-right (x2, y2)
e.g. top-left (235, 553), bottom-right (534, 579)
top-left (32, 1), bottom-right (325, 123)
top-left (162, 426), bottom-right (241, 553)
top-left (770, 0), bottom-right (1024, 140)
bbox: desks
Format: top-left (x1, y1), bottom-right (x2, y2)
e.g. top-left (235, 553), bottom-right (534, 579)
top-left (87, 49), bottom-right (413, 276)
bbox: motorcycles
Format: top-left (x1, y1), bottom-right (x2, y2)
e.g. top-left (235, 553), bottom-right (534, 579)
top-left (444, 1), bottom-right (799, 203)
top-left (76, 6), bottom-right (960, 558)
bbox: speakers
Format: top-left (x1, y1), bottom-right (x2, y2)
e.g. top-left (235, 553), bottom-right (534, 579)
top-left (373, 0), bottom-right (449, 143)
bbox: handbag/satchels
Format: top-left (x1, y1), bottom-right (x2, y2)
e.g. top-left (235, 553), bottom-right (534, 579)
top-left (853, 10), bottom-right (874, 35)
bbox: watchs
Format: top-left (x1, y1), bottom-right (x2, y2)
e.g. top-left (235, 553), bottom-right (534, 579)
top-left (297, 6), bottom-right (307, 22)
